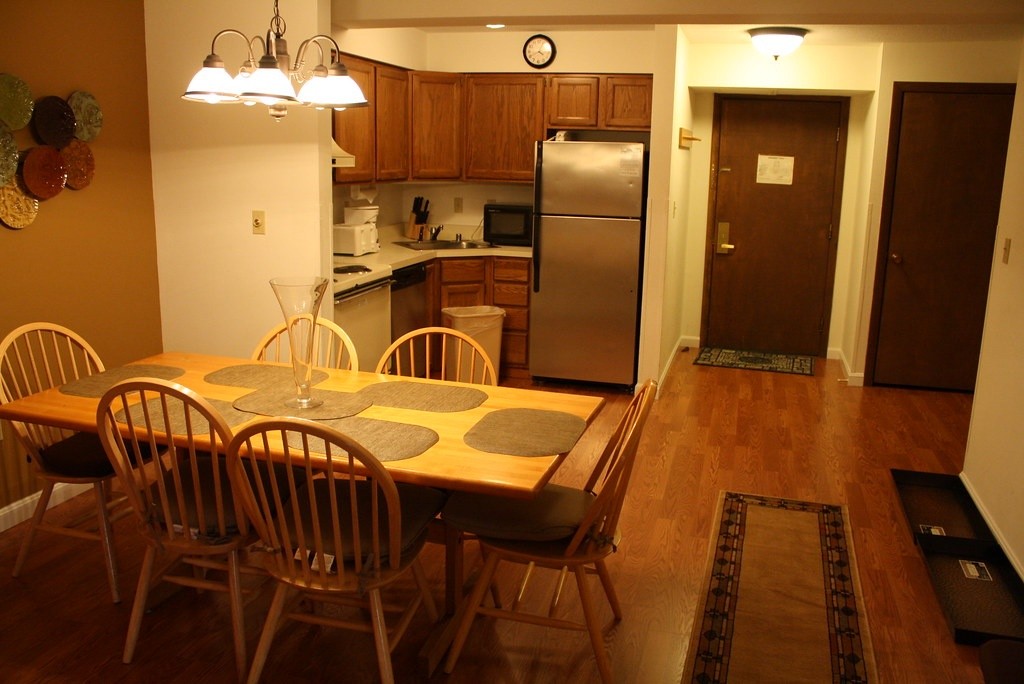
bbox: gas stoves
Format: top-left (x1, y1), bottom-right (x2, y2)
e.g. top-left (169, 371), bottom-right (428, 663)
top-left (334, 256), bottom-right (392, 299)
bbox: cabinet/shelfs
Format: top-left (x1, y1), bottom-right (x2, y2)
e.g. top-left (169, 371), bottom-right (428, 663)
top-left (331, 48), bottom-right (412, 186)
top-left (545, 72), bottom-right (653, 132)
top-left (462, 72), bottom-right (549, 184)
top-left (424, 257), bottom-right (530, 370)
top-left (408, 68), bottom-right (462, 184)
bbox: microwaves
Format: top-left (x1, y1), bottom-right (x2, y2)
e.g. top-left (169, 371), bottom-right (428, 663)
top-left (484, 205), bottom-right (533, 244)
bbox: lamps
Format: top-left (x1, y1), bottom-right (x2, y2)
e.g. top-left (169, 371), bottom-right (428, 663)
top-left (181, 0), bottom-right (369, 125)
top-left (745, 27), bottom-right (811, 62)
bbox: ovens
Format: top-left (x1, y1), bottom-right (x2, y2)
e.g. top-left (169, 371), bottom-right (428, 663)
top-left (335, 285), bottom-right (391, 374)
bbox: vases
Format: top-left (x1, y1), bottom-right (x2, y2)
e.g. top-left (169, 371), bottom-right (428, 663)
top-left (268, 274), bottom-right (330, 410)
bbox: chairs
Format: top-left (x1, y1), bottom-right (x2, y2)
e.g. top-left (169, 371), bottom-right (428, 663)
top-left (977, 638), bottom-right (1024, 684)
top-left (443, 378), bottom-right (659, 684)
top-left (223, 415), bottom-right (439, 684)
top-left (250, 312), bottom-right (360, 373)
top-left (374, 327), bottom-right (506, 611)
top-left (0, 321), bottom-right (168, 602)
top-left (96, 377), bottom-right (314, 684)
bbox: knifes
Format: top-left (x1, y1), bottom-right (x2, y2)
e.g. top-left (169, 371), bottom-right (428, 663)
top-left (412, 196), bottom-right (429, 224)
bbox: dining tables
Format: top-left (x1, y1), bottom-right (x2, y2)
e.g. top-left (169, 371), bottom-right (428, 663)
top-left (0, 352), bottom-right (606, 680)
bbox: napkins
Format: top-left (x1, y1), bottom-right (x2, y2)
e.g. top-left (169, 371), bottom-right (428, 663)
top-left (115, 394), bottom-right (256, 436)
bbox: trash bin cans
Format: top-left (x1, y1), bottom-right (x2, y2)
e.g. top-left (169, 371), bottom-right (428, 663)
top-left (441, 305), bottom-right (505, 385)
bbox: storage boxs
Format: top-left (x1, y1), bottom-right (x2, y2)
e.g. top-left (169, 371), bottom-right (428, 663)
top-left (889, 467), bottom-right (998, 546)
top-left (914, 531), bottom-right (1024, 648)
top-left (407, 212), bottom-right (427, 241)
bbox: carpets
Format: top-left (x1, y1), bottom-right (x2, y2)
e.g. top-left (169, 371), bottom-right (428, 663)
top-left (680, 488), bottom-right (867, 684)
top-left (692, 347), bottom-right (817, 378)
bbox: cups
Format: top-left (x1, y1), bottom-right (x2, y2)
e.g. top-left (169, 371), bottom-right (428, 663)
top-left (270, 275), bottom-right (331, 408)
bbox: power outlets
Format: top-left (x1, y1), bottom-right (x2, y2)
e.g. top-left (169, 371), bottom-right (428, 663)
top-left (486, 200), bottom-right (496, 204)
top-left (454, 197), bottom-right (464, 214)
top-left (251, 210), bottom-right (265, 235)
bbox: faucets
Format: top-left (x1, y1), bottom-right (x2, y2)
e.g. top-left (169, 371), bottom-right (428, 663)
top-left (430, 224), bottom-right (443, 240)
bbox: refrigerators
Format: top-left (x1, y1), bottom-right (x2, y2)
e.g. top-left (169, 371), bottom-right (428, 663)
top-left (528, 142), bottom-right (644, 386)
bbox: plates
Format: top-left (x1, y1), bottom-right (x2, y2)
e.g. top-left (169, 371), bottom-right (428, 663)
top-left (0, 72), bottom-right (103, 228)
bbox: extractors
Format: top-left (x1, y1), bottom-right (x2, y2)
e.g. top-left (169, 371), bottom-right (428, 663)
top-left (332, 136), bottom-right (355, 167)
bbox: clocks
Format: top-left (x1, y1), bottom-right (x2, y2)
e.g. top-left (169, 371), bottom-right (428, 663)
top-left (523, 34), bottom-right (557, 69)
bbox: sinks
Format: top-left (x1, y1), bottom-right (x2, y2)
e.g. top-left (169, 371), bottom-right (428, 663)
top-left (391, 239), bottom-right (502, 251)
